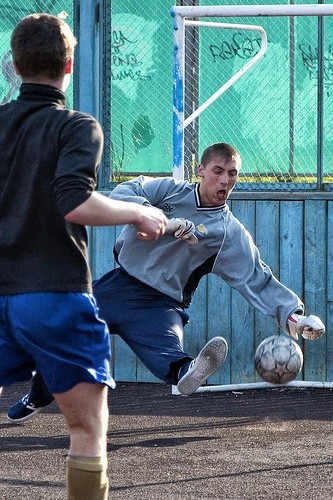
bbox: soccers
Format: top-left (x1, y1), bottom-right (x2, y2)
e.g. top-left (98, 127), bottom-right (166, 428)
top-left (254, 335), bottom-right (304, 385)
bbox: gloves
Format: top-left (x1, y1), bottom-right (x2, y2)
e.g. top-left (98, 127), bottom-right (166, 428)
top-left (287, 313), bottom-right (325, 340)
top-left (166, 218), bottom-right (198, 245)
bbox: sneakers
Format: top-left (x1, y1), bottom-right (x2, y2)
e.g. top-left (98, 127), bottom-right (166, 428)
top-left (176, 336), bottom-right (228, 396)
top-left (6, 393), bottom-right (43, 422)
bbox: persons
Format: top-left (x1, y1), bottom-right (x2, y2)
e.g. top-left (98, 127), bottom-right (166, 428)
top-left (0, 13), bottom-right (169, 500)
top-left (7, 142), bottom-right (325, 424)
